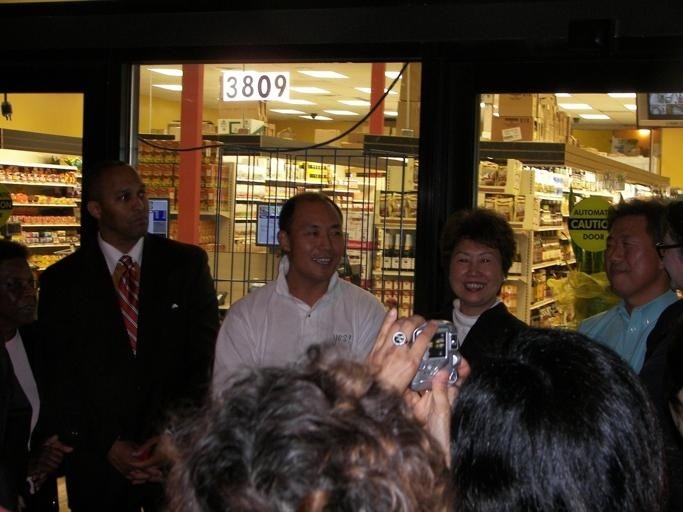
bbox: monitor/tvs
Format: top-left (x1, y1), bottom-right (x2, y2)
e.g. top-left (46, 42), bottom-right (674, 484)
top-left (634, 93), bottom-right (683, 130)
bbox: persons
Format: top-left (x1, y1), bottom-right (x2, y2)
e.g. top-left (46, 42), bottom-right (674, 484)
top-left (1, 241), bottom-right (76, 512)
top-left (447, 328), bottom-right (662, 511)
top-left (156, 306), bottom-right (472, 510)
top-left (577, 198), bottom-right (682, 419)
top-left (35, 162), bottom-right (220, 512)
top-left (214, 192), bottom-right (388, 405)
top-left (431, 209), bottom-right (527, 381)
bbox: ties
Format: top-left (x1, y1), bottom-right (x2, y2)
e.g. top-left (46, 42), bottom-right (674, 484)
top-left (117, 255), bottom-right (139, 354)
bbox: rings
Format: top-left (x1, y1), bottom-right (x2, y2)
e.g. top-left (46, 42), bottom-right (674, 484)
top-left (393, 332), bottom-right (408, 345)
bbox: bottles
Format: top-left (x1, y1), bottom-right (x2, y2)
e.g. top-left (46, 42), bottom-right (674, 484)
top-left (530, 304), bottom-right (558, 329)
top-left (537, 199), bottom-right (562, 226)
top-left (380, 232), bottom-right (413, 271)
top-left (533, 231), bottom-right (568, 267)
top-left (534, 167), bottom-right (595, 197)
top-left (531, 271), bottom-right (546, 305)
top-left (497, 283), bottom-right (516, 317)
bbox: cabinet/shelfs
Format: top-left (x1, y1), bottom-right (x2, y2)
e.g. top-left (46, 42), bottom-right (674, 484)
top-left (168, 160), bottom-right (236, 252)
top-left (372, 155), bottom-right (577, 331)
top-left (0, 162), bottom-right (82, 272)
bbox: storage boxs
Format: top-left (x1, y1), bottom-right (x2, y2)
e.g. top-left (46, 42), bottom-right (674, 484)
top-left (395, 62), bottom-right (573, 142)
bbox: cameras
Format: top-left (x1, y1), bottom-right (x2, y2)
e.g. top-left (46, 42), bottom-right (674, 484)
top-left (409, 319), bottom-right (461, 391)
